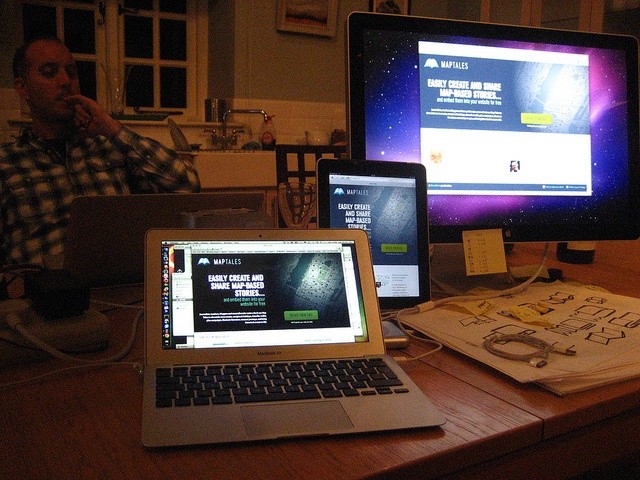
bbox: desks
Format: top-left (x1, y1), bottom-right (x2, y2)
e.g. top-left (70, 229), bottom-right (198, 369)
top-left (1, 231), bottom-right (639, 474)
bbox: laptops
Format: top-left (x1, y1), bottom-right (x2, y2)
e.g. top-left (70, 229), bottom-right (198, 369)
top-left (141, 228), bottom-right (447, 447)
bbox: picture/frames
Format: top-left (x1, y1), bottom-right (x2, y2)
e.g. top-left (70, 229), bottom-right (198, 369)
top-left (277, 1), bottom-right (340, 40)
top-left (370, 1), bottom-right (412, 16)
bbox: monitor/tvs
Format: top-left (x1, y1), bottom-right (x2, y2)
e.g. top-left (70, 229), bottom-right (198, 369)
top-left (345, 11), bottom-right (639, 240)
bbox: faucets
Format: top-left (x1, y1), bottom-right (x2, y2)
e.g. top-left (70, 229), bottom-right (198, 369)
top-left (222, 108), bottom-right (268, 136)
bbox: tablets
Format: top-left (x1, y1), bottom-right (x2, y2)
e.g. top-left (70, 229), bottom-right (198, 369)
top-left (317, 160), bottom-right (431, 311)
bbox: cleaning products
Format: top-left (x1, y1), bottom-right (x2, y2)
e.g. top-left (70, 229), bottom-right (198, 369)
top-left (258, 114), bottom-right (279, 151)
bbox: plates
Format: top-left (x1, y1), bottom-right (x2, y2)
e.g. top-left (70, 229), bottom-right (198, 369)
top-left (112, 112), bottom-right (169, 121)
top-left (167, 117), bottom-right (192, 152)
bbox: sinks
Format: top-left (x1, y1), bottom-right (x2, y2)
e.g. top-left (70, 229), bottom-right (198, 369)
top-left (178, 147), bottom-right (277, 158)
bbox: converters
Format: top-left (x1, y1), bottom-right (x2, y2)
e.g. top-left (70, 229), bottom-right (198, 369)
top-left (20, 268), bottom-right (92, 321)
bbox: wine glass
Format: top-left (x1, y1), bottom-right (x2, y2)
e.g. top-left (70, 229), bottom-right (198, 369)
top-left (277, 183), bottom-right (317, 228)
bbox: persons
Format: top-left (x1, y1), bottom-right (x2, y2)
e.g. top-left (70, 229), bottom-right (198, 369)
top-left (0, 36), bottom-right (201, 289)
top-left (511, 161), bottom-right (520, 172)
